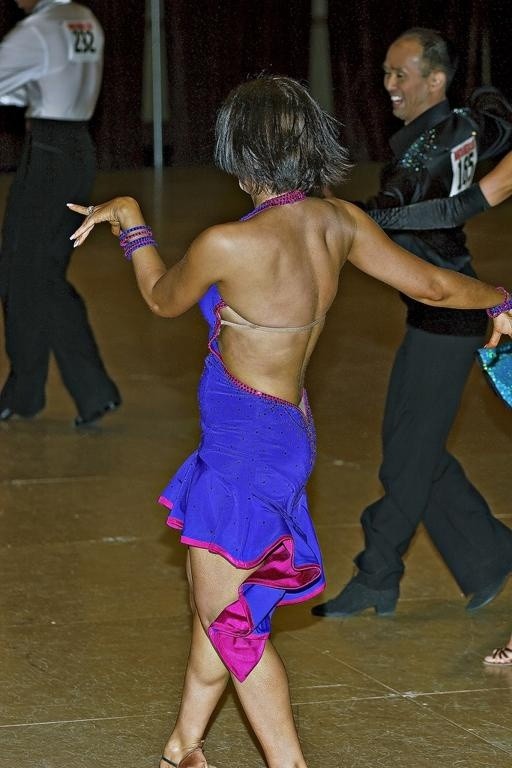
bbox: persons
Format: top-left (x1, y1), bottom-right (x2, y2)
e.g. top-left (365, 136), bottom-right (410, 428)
top-left (364, 149), bottom-right (511, 664)
top-left (63, 75), bottom-right (511, 768)
top-left (308, 28), bottom-right (512, 621)
top-left (2, 0), bottom-right (124, 428)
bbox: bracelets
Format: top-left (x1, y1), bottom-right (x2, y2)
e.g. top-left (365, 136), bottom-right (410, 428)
top-left (119, 224), bottom-right (158, 258)
top-left (484, 285), bottom-right (511, 320)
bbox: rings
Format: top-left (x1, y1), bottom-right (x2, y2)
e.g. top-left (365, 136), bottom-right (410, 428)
top-left (88, 206), bottom-right (94, 212)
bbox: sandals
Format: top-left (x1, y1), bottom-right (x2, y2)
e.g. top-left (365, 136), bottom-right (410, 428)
top-left (480, 645), bottom-right (512, 667)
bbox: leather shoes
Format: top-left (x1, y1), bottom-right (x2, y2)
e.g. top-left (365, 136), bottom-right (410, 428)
top-left (460, 568), bottom-right (506, 617)
top-left (310, 575), bottom-right (399, 621)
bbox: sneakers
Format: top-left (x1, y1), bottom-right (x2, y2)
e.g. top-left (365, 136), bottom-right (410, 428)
top-left (73, 397), bottom-right (121, 427)
top-left (0, 397), bottom-right (40, 420)
